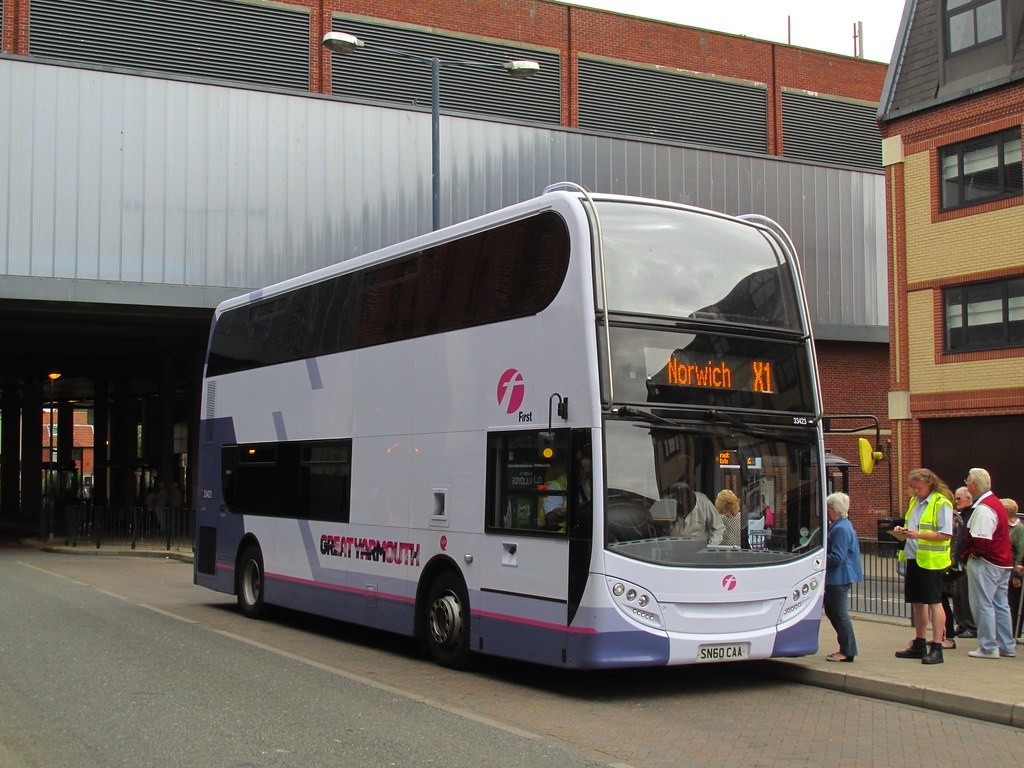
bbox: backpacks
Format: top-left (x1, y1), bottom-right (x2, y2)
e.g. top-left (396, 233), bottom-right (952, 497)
top-left (762, 502), bottom-right (774, 527)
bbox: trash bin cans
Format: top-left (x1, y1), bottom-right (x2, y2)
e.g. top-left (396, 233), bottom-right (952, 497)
top-left (877, 518), bottom-right (907, 557)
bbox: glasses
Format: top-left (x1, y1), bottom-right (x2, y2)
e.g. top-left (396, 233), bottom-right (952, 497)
top-left (954, 497), bottom-right (961, 500)
top-left (964, 479), bottom-right (972, 485)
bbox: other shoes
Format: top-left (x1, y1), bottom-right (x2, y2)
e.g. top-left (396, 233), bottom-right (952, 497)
top-left (926, 638), bottom-right (944, 645)
top-left (826, 652), bottom-right (854, 662)
top-left (954, 628), bottom-right (977, 638)
top-left (941, 639), bottom-right (956, 649)
top-left (968, 648), bottom-right (1000, 659)
top-left (922, 650), bottom-right (944, 664)
top-left (1000, 645), bottom-right (1015, 656)
top-left (895, 646), bottom-right (927, 658)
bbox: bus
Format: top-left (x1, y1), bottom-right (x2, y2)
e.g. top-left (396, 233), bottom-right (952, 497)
top-left (191, 184), bottom-right (887, 671)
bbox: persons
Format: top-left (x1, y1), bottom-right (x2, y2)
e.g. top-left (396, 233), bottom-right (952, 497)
top-left (894, 469), bottom-right (953, 664)
top-left (823, 492), bottom-right (864, 662)
top-left (543, 449), bottom-right (591, 532)
top-left (715, 489), bottom-right (741, 546)
top-left (954, 487), bottom-right (976, 638)
top-left (762, 495), bottom-right (773, 530)
top-left (961, 468), bottom-right (1016, 658)
top-left (999, 499), bottom-right (1024, 638)
top-left (926, 600), bottom-right (956, 649)
top-left (657, 483), bottom-right (726, 546)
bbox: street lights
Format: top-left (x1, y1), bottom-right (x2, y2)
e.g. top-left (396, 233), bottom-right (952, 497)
top-left (323, 32), bottom-right (542, 232)
